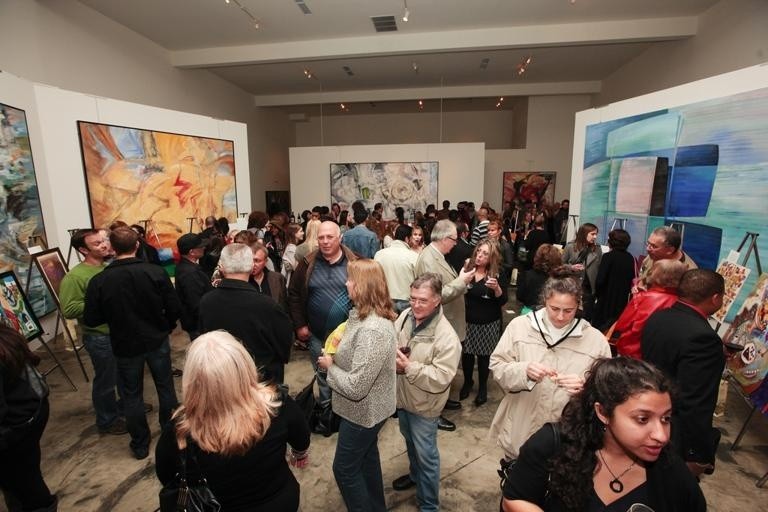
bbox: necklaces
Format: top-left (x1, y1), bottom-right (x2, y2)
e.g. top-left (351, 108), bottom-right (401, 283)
top-left (596, 445), bottom-right (640, 494)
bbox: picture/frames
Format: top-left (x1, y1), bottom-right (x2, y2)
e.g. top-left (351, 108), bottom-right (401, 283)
top-left (0, 103), bottom-right (58, 321)
top-left (77, 120), bottom-right (239, 278)
top-left (329, 160), bottom-right (439, 224)
top-left (0, 270), bottom-right (45, 346)
top-left (502, 170), bottom-right (556, 225)
top-left (30, 247), bottom-right (70, 319)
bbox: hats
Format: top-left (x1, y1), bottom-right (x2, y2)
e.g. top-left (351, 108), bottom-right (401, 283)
top-left (178, 233), bottom-right (209, 254)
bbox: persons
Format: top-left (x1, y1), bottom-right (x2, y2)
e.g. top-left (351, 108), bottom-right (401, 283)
top-left (242, 210), bottom-right (272, 251)
top-left (527, 213), bottom-right (552, 243)
top-left (488, 266), bottom-right (611, 470)
top-left (393, 271), bottom-right (463, 510)
top-left (413, 218), bottom-right (473, 430)
top-left (281, 222), bottom-right (304, 287)
top-left (245, 245), bottom-right (287, 313)
top-left (171, 234), bottom-right (213, 333)
top-left (297, 220), bottom-right (329, 263)
top-left (344, 212), bottom-right (381, 259)
top-left (384, 220), bottom-right (402, 248)
top-left (264, 218), bottom-right (288, 261)
top-left (489, 221), bottom-right (513, 281)
top-left (471, 207), bottom-right (492, 249)
top-left (78, 225), bottom-right (181, 460)
top-left (613, 259), bottom-right (689, 365)
top-left (1, 320), bottom-right (59, 511)
top-left (194, 242), bottom-right (295, 386)
top-left (374, 225), bottom-right (420, 319)
top-left (561, 221), bottom-right (602, 292)
top-left (155, 329), bottom-right (310, 512)
top-left (315, 258), bottom-right (397, 511)
top-left (460, 236), bottom-right (510, 406)
top-left (638, 268), bottom-right (726, 475)
top-left (412, 223), bottom-right (425, 250)
top-left (513, 241), bottom-right (565, 315)
top-left (631, 224), bottom-right (698, 298)
top-left (287, 220), bottom-right (360, 432)
top-left (58, 228), bottom-right (135, 435)
top-left (196, 215), bottom-right (219, 234)
top-left (298, 191), bottom-right (574, 218)
top-left (499, 354), bottom-right (708, 512)
top-left (583, 228), bottom-right (637, 331)
top-left (131, 223), bottom-right (159, 266)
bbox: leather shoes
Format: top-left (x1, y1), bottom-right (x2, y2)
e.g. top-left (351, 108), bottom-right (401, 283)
top-left (170, 365), bottom-right (182, 374)
top-left (115, 401), bottom-right (153, 413)
top-left (394, 474), bottom-right (416, 488)
top-left (443, 398), bottom-right (458, 407)
top-left (98, 419), bottom-right (127, 433)
top-left (436, 416), bottom-right (452, 430)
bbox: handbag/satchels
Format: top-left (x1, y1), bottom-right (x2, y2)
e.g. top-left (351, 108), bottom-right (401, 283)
top-left (155, 424), bottom-right (222, 512)
top-left (295, 361), bottom-right (336, 437)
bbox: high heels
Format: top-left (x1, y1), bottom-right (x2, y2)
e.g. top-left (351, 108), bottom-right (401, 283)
top-left (460, 381), bottom-right (472, 398)
top-left (476, 388), bottom-right (486, 405)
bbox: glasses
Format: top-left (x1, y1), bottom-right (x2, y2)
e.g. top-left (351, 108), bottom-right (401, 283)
top-left (317, 234), bottom-right (342, 241)
top-left (644, 241), bottom-right (669, 251)
top-left (448, 236), bottom-right (457, 243)
top-left (477, 250), bottom-right (490, 255)
top-left (408, 297), bottom-right (437, 303)
top-left (252, 259), bottom-right (265, 263)
